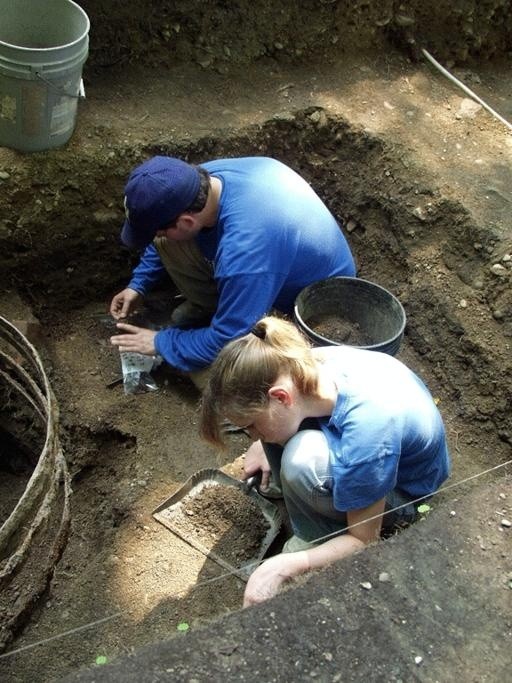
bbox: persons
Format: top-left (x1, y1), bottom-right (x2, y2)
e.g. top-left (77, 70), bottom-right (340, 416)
top-left (109, 155), bottom-right (357, 373)
top-left (191, 318), bottom-right (448, 610)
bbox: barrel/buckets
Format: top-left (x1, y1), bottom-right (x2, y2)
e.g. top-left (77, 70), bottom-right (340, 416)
top-left (293, 276), bottom-right (408, 357)
top-left (0, 0), bottom-right (91, 153)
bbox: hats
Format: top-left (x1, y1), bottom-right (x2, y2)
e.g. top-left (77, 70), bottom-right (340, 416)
top-left (120, 154), bottom-right (200, 250)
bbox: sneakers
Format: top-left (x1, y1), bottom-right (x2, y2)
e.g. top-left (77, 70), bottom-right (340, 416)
top-left (171, 300), bottom-right (216, 325)
top-left (259, 476), bottom-right (285, 499)
top-left (281, 533), bottom-right (318, 553)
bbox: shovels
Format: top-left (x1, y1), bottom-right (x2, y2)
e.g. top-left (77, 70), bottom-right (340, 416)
top-left (150, 463), bottom-right (285, 585)
